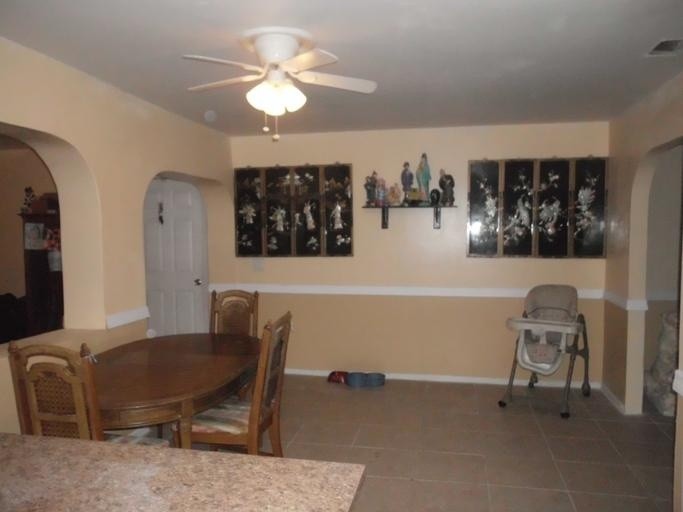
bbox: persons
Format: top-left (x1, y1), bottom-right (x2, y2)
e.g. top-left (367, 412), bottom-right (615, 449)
top-left (365, 152), bottom-right (454, 207)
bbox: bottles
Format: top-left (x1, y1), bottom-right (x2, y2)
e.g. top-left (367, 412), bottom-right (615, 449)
top-left (375, 179), bottom-right (385, 207)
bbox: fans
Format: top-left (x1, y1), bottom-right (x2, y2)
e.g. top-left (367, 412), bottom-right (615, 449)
top-left (181, 26), bottom-right (379, 95)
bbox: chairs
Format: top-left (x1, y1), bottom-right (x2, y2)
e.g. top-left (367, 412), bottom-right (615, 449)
top-left (207, 288), bottom-right (260, 402)
top-left (172, 310), bottom-right (292, 459)
top-left (495, 283), bottom-right (592, 419)
top-left (6, 339), bottom-right (170, 450)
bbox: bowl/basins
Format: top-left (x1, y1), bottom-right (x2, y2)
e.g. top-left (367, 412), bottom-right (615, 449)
top-left (345, 372), bottom-right (367, 387)
top-left (366, 372), bottom-right (385, 386)
top-left (327, 370), bottom-right (348, 384)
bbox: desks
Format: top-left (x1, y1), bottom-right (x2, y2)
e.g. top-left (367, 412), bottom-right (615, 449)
top-left (63, 333), bottom-right (265, 449)
top-left (0, 431), bottom-right (366, 512)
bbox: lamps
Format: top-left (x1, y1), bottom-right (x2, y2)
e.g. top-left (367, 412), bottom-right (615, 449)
top-left (245, 71), bottom-right (306, 143)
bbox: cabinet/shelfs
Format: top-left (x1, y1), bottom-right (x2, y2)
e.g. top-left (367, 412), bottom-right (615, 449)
top-left (18, 212), bottom-right (62, 331)
